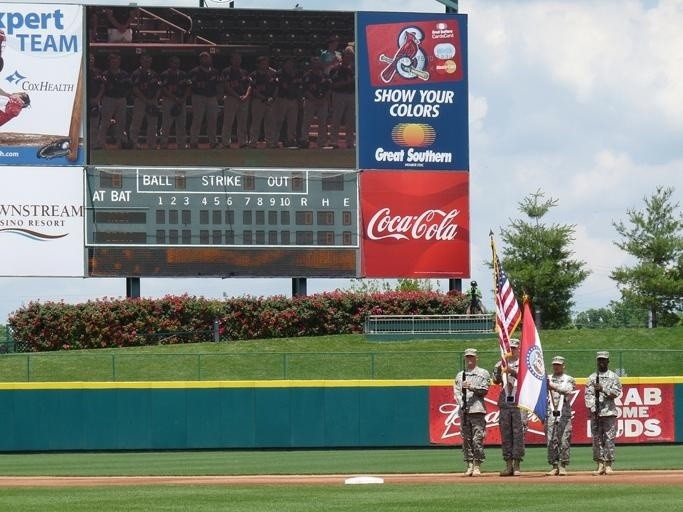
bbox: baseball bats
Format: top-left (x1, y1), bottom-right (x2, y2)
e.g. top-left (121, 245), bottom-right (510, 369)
top-left (380, 55), bottom-right (429, 80)
top-left (67, 60), bottom-right (83, 161)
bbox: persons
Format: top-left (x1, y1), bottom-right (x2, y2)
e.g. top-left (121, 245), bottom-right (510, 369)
top-left (453, 348), bottom-right (491, 477)
top-left (86, 6), bottom-right (356, 151)
top-left (584, 350), bottom-right (622, 474)
top-left (493, 338), bottom-right (528, 477)
top-left (544, 356), bottom-right (575, 476)
top-left (0, 29), bottom-right (24, 125)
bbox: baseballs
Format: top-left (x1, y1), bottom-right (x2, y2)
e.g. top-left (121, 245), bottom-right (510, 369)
top-left (62, 142), bottom-right (69, 150)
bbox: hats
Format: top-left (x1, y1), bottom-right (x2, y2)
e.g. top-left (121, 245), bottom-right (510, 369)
top-left (168, 101), bottom-right (185, 117)
top-left (508, 339), bottom-right (521, 348)
top-left (463, 348), bottom-right (479, 358)
top-left (144, 103), bottom-right (160, 118)
top-left (596, 350), bottom-right (611, 359)
top-left (551, 355), bottom-right (566, 365)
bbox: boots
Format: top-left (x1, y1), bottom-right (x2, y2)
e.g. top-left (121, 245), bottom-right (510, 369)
top-left (594, 460), bottom-right (603, 475)
top-left (557, 462), bottom-right (567, 476)
top-left (473, 459), bottom-right (483, 478)
top-left (605, 461), bottom-right (613, 475)
top-left (546, 464), bottom-right (559, 476)
top-left (499, 460), bottom-right (513, 476)
top-left (463, 461), bottom-right (474, 477)
top-left (514, 460), bottom-right (522, 476)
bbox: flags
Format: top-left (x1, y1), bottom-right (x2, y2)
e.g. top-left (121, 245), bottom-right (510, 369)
top-left (512, 292), bottom-right (548, 425)
top-left (490, 234), bottom-right (522, 388)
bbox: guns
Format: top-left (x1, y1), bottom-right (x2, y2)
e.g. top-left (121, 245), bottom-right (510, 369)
top-left (594, 369), bottom-right (601, 430)
top-left (461, 359), bottom-right (467, 426)
